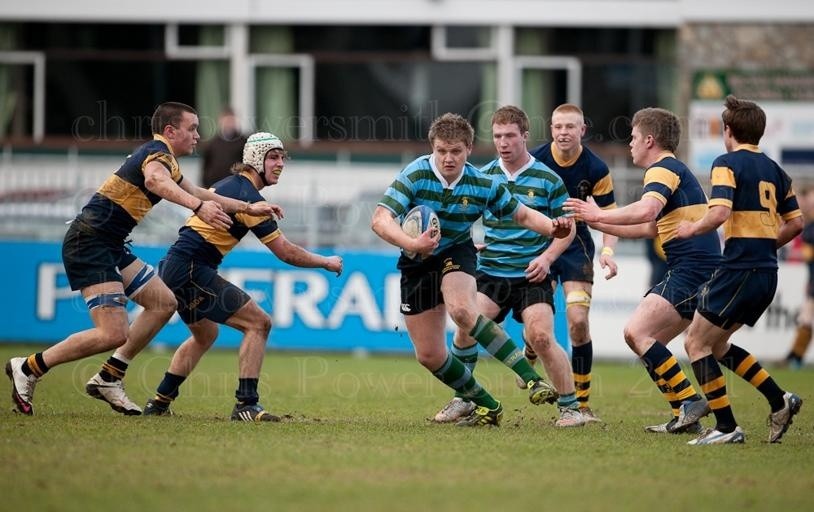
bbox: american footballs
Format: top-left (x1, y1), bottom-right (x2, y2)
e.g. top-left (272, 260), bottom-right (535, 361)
top-left (400, 206), bottom-right (441, 262)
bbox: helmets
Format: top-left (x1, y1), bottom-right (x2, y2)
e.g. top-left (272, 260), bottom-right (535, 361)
top-left (243, 132), bottom-right (284, 174)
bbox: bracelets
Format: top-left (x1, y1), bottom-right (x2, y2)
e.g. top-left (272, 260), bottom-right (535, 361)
top-left (245, 200), bottom-right (250, 213)
top-left (194, 200), bottom-right (203, 213)
top-left (601, 247), bottom-right (614, 255)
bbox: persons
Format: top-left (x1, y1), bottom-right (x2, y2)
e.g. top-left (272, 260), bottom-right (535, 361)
top-left (6, 101), bottom-right (285, 420)
top-left (676, 94), bottom-right (802, 447)
top-left (201, 106), bottom-right (251, 190)
top-left (779, 181), bottom-right (813, 366)
top-left (433, 106), bottom-right (587, 428)
top-left (516, 103), bottom-right (620, 425)
top-left (146, 132), bottom-right (343, 421)
top-left (370, 113), bottom-right (572, 432)
top-left (563, 107), bottom-right (723, 433)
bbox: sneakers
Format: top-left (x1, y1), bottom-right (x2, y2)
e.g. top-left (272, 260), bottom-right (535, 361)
top-left (556, 401), bottom-right (602, 427)
top-left (5, 357), bottom-right (42, 415)
top-left (231, 404), bottom-right (281, 422)
top-left (645, 398), bottom-right (712, 434)
top-left (435, 397), bottom-right (477, 422)
top-left (143, 399), bottom-right (182, 417)
top-left (455, 400), bottom-right (504, 427)
top-left (86, 373), bottom-right (142, 416)
top-left (687, 425), bottom-right (744, 445)
top-left (767, 391), bottom-right (802, 443)
top-left (516, 345), bottom-right (559, 405)
